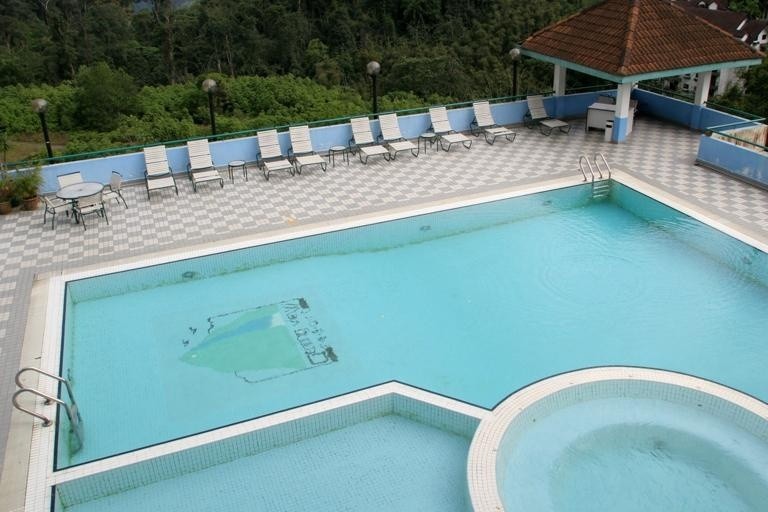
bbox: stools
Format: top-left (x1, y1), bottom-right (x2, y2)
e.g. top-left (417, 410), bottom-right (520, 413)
top-left (228, 160), bottom-right (248, 184)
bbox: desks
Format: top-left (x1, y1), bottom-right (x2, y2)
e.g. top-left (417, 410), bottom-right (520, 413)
top-left (55, 181), bottom-right (103, 224)
top-left (329, 145), bottom-right (349, 168)
top-left (585, 102), bottom-right (633, 136)
top-left (419, 133), bottom-right (438, 154)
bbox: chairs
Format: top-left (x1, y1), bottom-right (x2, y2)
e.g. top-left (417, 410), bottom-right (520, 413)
top-left (57, 172), bottom-right (87, 216)
top-left (186, 139), bottom-right (223, 191)
top-left (288, 125), bottom-right (328, 174)
top-left (523, 95), bottom-right (570, 136)
top-left (37, 193), bottom-right (79, 230)
top-left (425, 107), bottom-right (472, 152)
top-left (376, 113), bottom-right (419, 160)
top-left (470, 102), bottom-right (516, 145)
top-left (144, 145), bottom-right (178, 199)
top-left (598, 96), bottom-right (637, 127)
top-left (256, 129), bottom-right (295, 181)
top-left (72, 191), bottom-right (109, 231)
top-left (102, 171), bottom-right (130, 211)
top-left (349, 117), bottom-right (391, 164)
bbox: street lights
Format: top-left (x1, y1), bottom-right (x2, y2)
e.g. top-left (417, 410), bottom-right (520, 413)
top-left (506, 47), bottom-right (521, 102)
top-left (365, 59), bottom-right (382, 119)
top-left (201, 78), bottom-right (219, 142)
top-left (31, 99), bottom-right (56, 164)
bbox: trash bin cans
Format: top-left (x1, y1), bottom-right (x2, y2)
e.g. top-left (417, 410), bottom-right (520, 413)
top-left (605, 120), bottom-right (613, 142)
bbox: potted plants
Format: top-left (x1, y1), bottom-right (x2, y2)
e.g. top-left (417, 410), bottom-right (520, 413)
top-left (0, 172), bottom-right (15, 213)
top-left (12, 159), bottom-right (44, 209)
